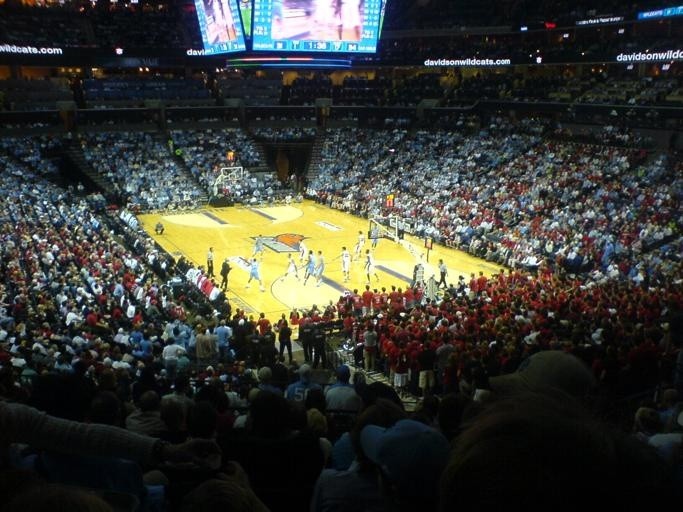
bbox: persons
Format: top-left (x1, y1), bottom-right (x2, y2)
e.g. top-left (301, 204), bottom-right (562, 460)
top-left (278, 252), bottom-right (300, 282)
top-left (342, 207), bottom-right (681, 511)
top-left (245, 257), bottom-right (264, 292)
top-left (1, 1), bottom-right (653, 211)
top-left (331, 247), bottom-right (352, 283)
top-left (303, 249), bottom-right (314, 286)
top-left (1, 203), bottom-right (250, 511)
top-left (247, 233), bottom-right (264, 261)
top-left (297, 239), bottom-right (306, 265)
top-left (249, 298), bottom-right (340, 511)
top-left (314, 250), bottom-right (324, 288)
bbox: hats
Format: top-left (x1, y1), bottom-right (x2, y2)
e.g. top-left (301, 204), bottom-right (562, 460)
top-left (258, 367), bottom-right (272, 380)
top-left (333, 365), bottom-right (350, 379)
top-left (359, 418), bottom-right (450, 481)
top-left (488, 351), bottom-right (590, 410)
top-left (295, 364), bottom-right (312, 376)
